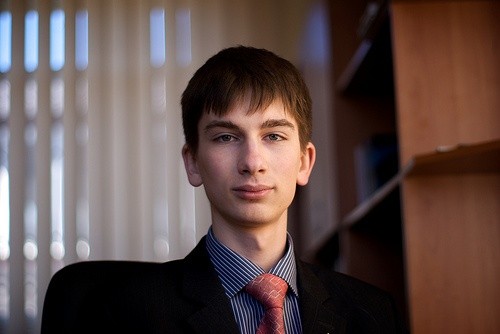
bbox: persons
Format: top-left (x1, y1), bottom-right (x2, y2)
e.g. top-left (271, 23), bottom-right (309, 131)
top-left (79, 46), bottom-right (410, 334)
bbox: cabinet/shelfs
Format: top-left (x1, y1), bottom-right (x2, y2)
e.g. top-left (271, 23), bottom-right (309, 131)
top-left (288, 0), bottom-right (500, 334)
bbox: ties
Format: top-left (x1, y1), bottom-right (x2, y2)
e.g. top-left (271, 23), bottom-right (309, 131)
top-left (244, 273), bottom-right (290, 334)
